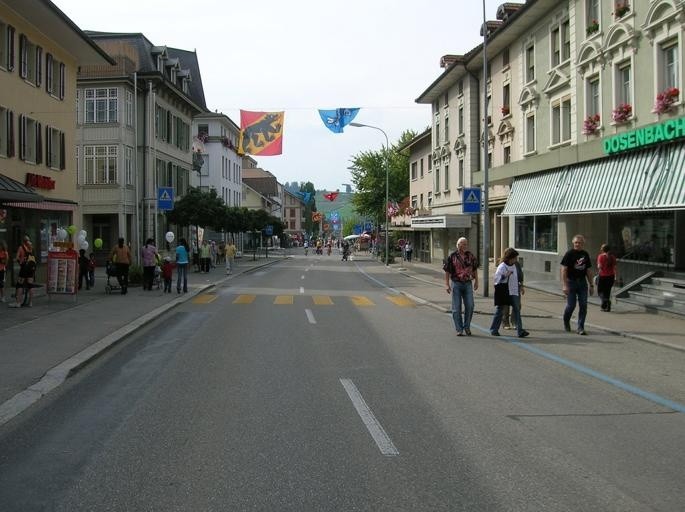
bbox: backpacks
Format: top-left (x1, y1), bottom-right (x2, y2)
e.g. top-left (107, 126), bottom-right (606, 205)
top-left (21, 245), bottom-right (37, 273)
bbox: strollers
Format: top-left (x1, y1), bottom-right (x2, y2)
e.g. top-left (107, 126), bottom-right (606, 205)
top-left (104, 258), bottom-right (127, 295)
top-left (150, 262), bottom-right (163, 291)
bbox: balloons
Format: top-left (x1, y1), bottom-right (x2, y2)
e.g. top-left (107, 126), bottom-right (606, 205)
top-left (166, 231), bottom-right (175, 243)
top-left (59, 227), bottom-right (104, 252)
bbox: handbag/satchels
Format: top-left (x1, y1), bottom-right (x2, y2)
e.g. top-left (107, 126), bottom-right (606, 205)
top-left (493, 281), bottom-right (511, 307)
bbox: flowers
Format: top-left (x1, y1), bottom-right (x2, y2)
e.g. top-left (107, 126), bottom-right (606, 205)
top-left (651, 87), bottom-right (681, 115)
top-left (612, 102), bottom-right (633, 123)
top-left (501, 106), bottom-right (509, 115)
top-left (584, 114), bottom-right (600, 135)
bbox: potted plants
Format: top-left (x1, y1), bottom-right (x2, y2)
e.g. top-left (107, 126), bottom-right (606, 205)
top-left (130, 264), bottom-right (142, 285)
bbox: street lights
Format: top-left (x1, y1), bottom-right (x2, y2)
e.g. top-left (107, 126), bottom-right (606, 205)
top-left (348, 121), bottom-right (392, 269)
top-left (194, 149), bottom-right (204, 250)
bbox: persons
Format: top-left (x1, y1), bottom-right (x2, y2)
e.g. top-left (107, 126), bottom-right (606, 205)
top-left (560, 235), bottom-right (595, 334)
top-left (7, 235), bottom-right (36, 308)
top-left (595, 244), bottom-right (619, 312)
top-left (0, 240), bottom-right (9, 302)
top-left (302, 237), bottom-right (354, 262)
top-left (443, 237), bottom-right (479, 335)
top-left (77, 249), bottom-right (90, 290)
top-left (110, 238), bottom-right (237, 294)
top-left (503, 262), bottom-right (524, 330)
top-left (405, 241), bottom-right (413, 262)
top-left (89, 253), bottom-right (97, 287)
top-left (491, 248), bottom-right (530, 338)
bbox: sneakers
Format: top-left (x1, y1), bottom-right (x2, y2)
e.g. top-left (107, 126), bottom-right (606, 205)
top-left (465, 328), bottom-right (471, 336)
top-left (77, 284), bottom-right (188, 295)
top-left (23, 304), bottom-right (32, 307)
top-left (563, 321), bottom-right (571, 332)
top-left (576, 328), bottom-right (585, 335)
top-left (456, 330), bottom-right (462, 336)
top-left (518, 331), bottom-right (529, 338)
top-left (7, 302), bottom-right (22, 308)
top-left (491, 331), bottom-right (500, 336)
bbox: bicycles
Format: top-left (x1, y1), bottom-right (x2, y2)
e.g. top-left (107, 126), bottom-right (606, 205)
top-left (303, 246), bottom-right (351, 263)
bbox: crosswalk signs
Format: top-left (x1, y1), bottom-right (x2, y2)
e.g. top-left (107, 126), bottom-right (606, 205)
top-left (157, 186), bottom-right (174, 209)
top-left (461, 188), bottom-right (482, 213)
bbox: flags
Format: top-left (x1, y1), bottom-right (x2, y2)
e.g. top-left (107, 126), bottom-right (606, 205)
top-left (238, 109), bottom-right (285, 156)
